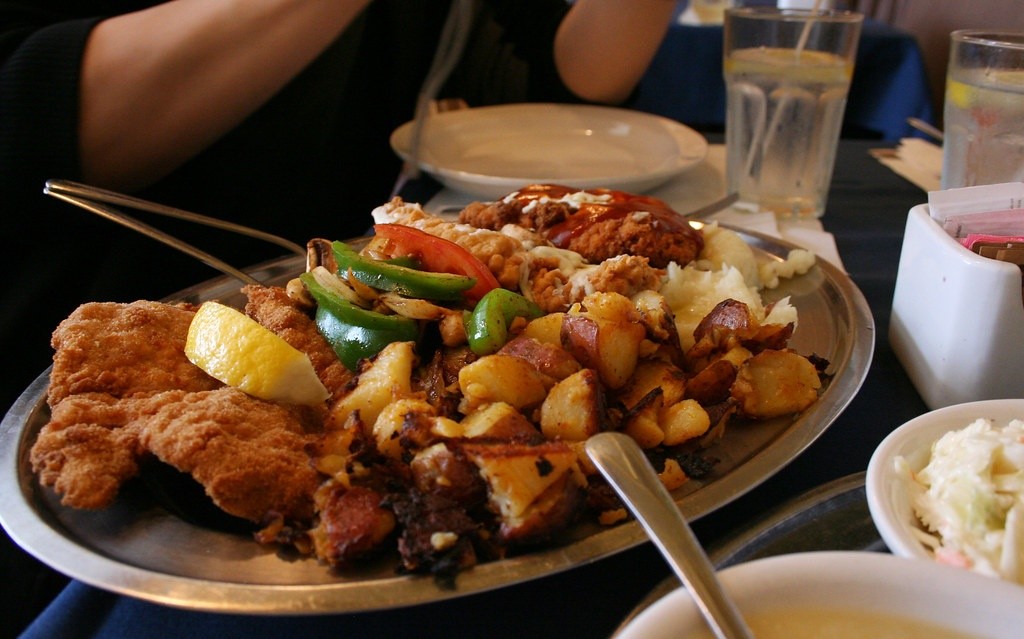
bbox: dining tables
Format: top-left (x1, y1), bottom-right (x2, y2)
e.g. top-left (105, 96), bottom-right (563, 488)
top-left (17, 135), bottom-right (928, 639)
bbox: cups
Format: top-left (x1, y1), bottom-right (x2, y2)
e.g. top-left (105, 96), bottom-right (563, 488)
top-left (941, 29), bottom-right (1024, 188)
top-left (720, 5), bottom-right (865, 220)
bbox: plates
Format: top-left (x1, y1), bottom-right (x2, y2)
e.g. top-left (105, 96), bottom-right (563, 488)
top-left (389, 102), bottom-right (707, 202)
top-left (0, 219), bottom-right (876, 615)
top-left (866, 397), bottom-right (1024, 560)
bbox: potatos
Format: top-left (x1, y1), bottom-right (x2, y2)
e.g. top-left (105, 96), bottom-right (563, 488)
top-left (305, 294), bottom-right (825, 576)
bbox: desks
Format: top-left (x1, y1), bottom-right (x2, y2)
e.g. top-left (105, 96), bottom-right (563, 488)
top-left (632, 1), bottom-right (934, 140)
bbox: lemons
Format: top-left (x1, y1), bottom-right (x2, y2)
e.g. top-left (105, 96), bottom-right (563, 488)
top-left (184, 300), bottom-right (333, 408)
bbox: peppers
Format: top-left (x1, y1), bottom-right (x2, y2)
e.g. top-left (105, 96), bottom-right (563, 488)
top-left (299, 220), bottom-right (547, 369)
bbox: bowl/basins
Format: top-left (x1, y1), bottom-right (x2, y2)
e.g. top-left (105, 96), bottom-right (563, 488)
top-left (615, 551), bottom-right (1024, 639)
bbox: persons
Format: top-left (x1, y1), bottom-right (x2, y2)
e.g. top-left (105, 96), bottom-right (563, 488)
top-left (0, 0), bottom-right (676, 420)
